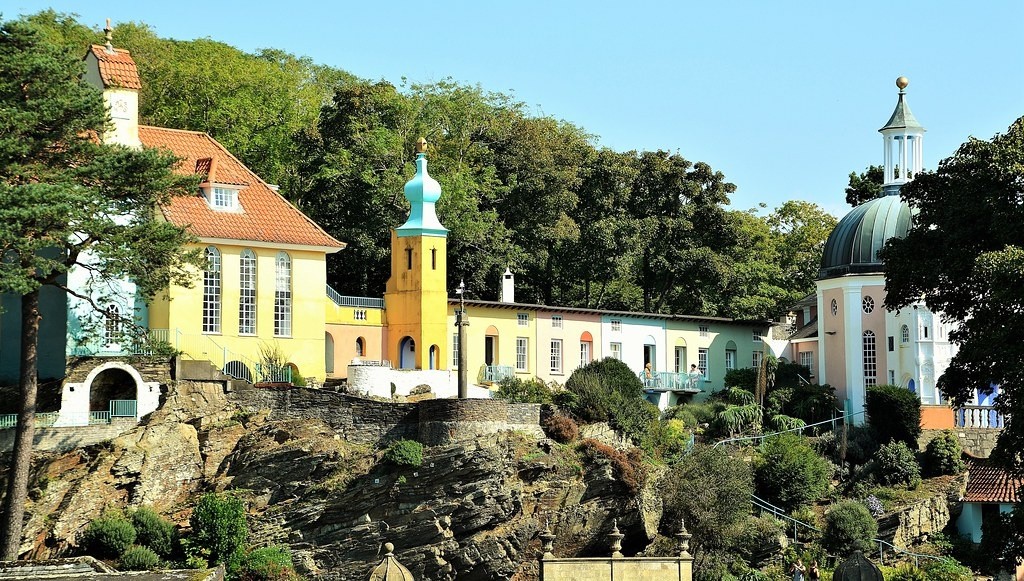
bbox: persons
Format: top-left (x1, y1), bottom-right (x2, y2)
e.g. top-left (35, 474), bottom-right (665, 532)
top-left (645, 363), bottom-right (664, 385)
top-left (808, 560), bottom-right (820, 581)
top-left (689, 364), bottom-right (703, 386)
top-left (790, 558), bottom-right (807, 581)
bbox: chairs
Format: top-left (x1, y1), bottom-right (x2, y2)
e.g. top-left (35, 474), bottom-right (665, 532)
top-left (692, 381), bottom-right (698, 387)
top-left (642, 370), bottom-right (653, 387)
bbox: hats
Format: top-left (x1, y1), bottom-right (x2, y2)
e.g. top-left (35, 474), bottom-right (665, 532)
top-left (646, 362), bottom-right (652, 367)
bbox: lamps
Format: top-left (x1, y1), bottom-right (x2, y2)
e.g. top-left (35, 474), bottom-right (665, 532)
top-left (825, 332), bottom-right (836, 336)
